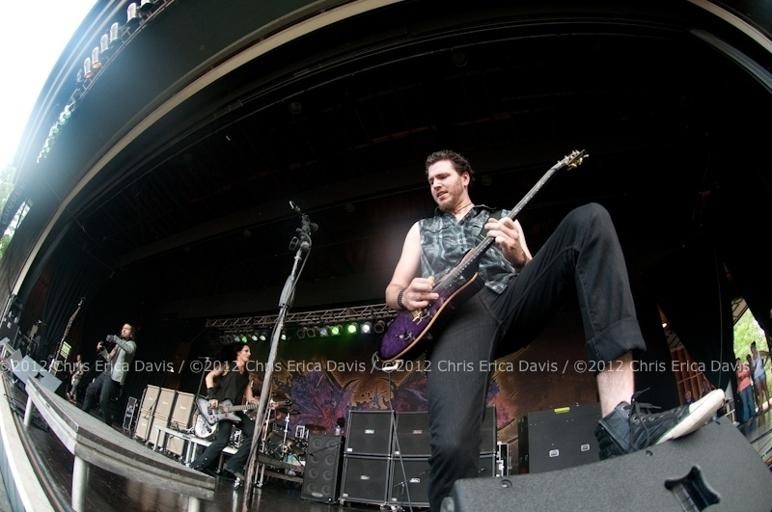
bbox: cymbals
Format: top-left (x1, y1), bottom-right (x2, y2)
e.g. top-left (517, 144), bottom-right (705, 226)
top-left (304, 424), bottom-right (326, 431)
top-left (279, 408), bottom-right (301, 415)
top-left (271, 393), bottom-right (289, 402)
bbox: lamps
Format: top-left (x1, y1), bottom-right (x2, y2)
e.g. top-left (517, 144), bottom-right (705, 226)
top-left (220, 318), bottom-right (393, 344)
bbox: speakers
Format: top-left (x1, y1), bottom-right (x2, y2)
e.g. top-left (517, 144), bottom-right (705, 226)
top-left (517, 402), bottom-right (602, 473)
top-left (121, 396), bottom-right (138, 430)
top-left (438, 416), bottom-right (772, 512)
top-left (2, 348), bottom-right (69, 401)
top-left (338, 404), bottom-right (497, 509)
top-left (299, 433), bottom-right (346, 505)
top-left (0, 315), bottom-right (20, 348)
top-left (134, 383), bottom-right (196, 458)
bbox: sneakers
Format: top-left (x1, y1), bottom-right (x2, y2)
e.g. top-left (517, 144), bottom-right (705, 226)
top-left (223, 463), bottom-right (245, 481)
top-left (594, 388), bottom-right (725, 460)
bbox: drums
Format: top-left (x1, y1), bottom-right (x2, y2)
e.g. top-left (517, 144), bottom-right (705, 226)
top-left (190, 406), bottom-right (219, 440)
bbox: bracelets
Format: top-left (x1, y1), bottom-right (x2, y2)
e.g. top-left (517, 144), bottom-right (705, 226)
top-left (208, 388), bottom-right (215, 399)
top-left (396, 286), bottom-right (407, 311)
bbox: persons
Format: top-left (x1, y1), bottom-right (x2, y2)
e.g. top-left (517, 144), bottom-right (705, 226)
top-left (682, 390), bottom-right (696, 404)
top-left (748, 340), bottom-right (770, 417)
top-left (66, 353), bottom-right (83, 404)
top-left (185, 341), bottom-right (280, 484)
top-left (734, 357), bottom-right (756, 423)
top-left (81, 322), bottom-right (137, 428)
top-left (384, 148), bottom-right (727, 510)
top-left (701, 378), bottom-right (716, 397)
top-left (745, 354), bottom-right (755, 391)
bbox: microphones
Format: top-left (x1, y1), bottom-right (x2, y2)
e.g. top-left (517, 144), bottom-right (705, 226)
top-left (81, 296), bottom-right (86, 302)
top-left (290, 199), bottom-right (319, 232)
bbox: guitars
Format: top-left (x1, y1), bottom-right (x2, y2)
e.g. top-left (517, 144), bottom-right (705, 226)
top-left (379, 149), bottom-right (588, 366)
top-left (196, 394), bottom-right (296, 426)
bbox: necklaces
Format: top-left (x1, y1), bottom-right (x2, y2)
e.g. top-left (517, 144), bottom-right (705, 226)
top-left (453, 202), bottom-right (474, 213)
